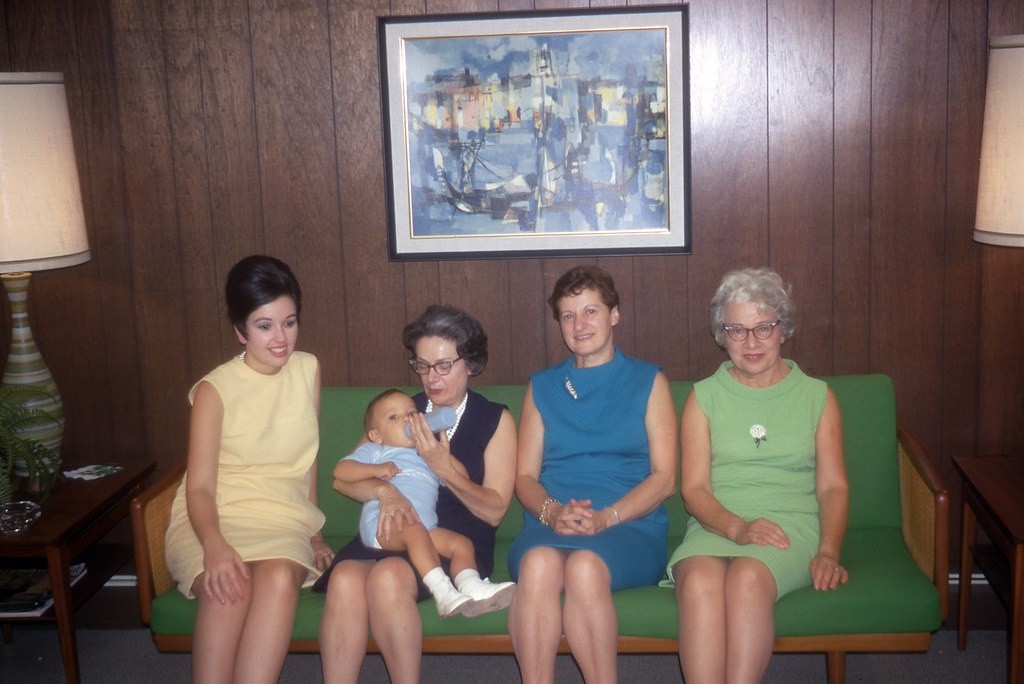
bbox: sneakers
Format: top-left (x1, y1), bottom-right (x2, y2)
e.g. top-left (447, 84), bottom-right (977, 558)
top-left (459, 577), bottom-right (517, 617)
top-left (436, 589), bottom-right (472, 618)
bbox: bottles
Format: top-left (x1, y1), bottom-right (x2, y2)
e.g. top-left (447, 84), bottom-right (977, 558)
top-left (405, 406), bottom-right (457, 439)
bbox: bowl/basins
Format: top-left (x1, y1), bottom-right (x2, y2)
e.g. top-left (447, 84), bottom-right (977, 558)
top-left (0, 501), bottom-right (41, 535)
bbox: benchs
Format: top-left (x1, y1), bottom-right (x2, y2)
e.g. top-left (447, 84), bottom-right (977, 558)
top-left (129, 372), bottom-right (949, 684)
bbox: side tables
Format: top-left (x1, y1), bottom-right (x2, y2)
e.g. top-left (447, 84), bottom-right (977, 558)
top-left (951, 455), bottom-right (1024, 684)
top-left (0, 458), bottom-right (163, 684)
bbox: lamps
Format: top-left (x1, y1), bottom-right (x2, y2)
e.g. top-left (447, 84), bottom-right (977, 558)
top-left (971, 32), bottom-right (1024, 248)
top-left (0, 71), bottom-right (92, 490)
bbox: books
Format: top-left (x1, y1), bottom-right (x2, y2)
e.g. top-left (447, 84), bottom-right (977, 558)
top-left (0, 562), bottom-right (86, 618)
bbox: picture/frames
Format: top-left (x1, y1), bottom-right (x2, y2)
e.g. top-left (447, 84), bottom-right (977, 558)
top-left (377, 3), bottom-right (695, 263)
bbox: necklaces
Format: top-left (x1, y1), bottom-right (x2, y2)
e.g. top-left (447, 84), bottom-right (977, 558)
top-left (424, 393), bottom-right (467, 441)
top-left (239, 351), bottom-right (246, 363)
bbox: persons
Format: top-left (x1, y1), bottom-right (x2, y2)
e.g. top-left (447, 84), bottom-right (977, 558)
top-left (312, 306), bottom-right (517, 684)
top-left (163, 254), bottom-right (336, 684)
top-left (334, 389), bottom-right (517, 617)
top-left (659, 267), bottom-right (849, 684)
top-left (507, 265), bottom-right (677, 684)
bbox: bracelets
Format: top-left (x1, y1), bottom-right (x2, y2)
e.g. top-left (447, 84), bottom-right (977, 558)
top-left (608, 506), bottom-right (620, 524)
top-left (538, 497), bottom-right (560, 526)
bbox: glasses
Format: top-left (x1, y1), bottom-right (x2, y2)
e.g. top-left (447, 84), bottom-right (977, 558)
top-left (408, 355), bottom-right (461, 375)
top-left (722, 320), bottom-right (781, 342)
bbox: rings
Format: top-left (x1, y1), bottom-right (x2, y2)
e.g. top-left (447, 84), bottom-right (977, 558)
top-left (576, 520), bottom-right (581, 525)
top-left (834, 568), bottom-right (840, 571)
top-left (384, 513), bottom-right (393, 517)
top-left (324, 553), bottom-right (330, 558)
